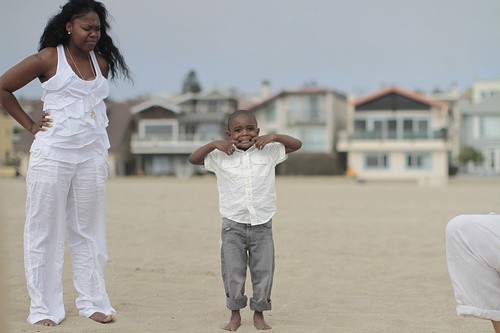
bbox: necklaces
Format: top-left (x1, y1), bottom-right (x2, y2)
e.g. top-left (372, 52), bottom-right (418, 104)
top-left (64, 42), bottom-right (99, 118)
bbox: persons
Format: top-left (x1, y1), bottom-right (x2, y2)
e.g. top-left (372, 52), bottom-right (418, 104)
top-left (187, 111), bottom-right (302, 330)
top-left (0, 0), bottom-right (135, 327)
top-left (445, 213), bottom-right (500, 331)
